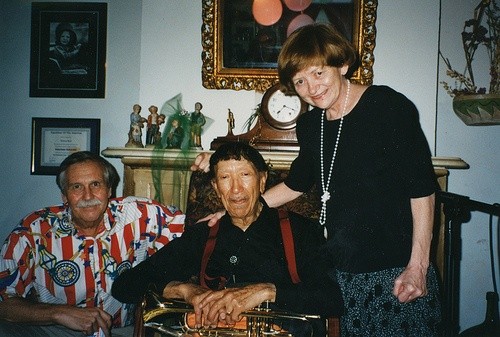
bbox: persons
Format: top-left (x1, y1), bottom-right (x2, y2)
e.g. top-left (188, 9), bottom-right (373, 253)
top-left (48, 22), bottom-right (82, 87)
top-left (0, 151), bottom-right (186, 337)
top-left (124, 102), bottom-right (206, 150)
top-left (196, 24), bottom-right (442, 336)
top-left (112, 142), bottom-right (345, 337)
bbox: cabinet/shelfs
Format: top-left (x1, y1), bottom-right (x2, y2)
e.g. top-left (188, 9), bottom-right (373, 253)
top-left (101, 146), bottom-right (470, 337)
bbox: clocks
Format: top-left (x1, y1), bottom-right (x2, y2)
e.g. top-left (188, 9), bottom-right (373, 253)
top-left (209, 83), bottom-right (310, 151)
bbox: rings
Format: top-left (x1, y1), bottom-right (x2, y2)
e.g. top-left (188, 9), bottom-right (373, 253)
top-left (225, 310), bottom-right (232, 316)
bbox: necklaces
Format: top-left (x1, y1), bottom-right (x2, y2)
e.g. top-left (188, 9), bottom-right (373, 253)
top-left (303, 78), bottom-right (351, 225)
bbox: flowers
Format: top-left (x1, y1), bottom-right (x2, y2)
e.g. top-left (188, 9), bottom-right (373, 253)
top-left (439, 0), bottom-right (500, 98)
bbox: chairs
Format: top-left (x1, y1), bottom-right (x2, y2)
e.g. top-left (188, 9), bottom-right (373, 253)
top-left (132, 293), bottom-right (344, 337)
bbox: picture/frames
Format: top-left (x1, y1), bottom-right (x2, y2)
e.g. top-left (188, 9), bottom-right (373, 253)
top-left (29, 0), bottom-right (108, 99)
top-left (30, 117), bottom-right (100, 176)
top-left (201, 0), bottom-right (378, 92)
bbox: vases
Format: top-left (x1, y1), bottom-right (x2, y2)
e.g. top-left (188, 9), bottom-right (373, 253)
top-left (452, 95), bottom-right (500, 126)
